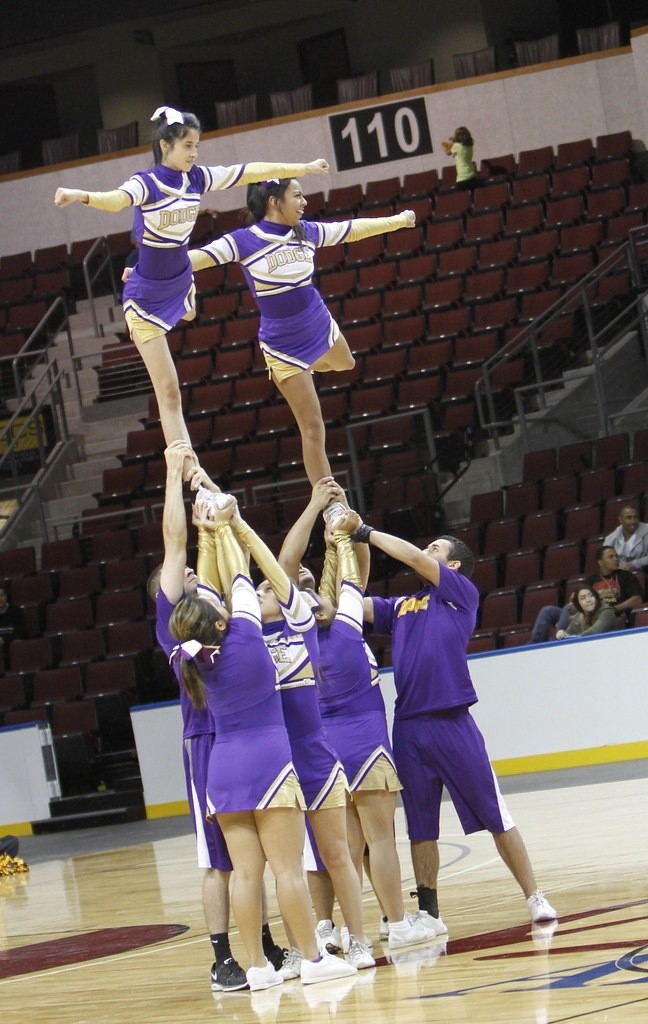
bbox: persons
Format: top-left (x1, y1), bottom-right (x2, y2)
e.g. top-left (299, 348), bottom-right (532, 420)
top-left (439, 127), bottom-right (480, 192)
top-left (527, 547), bottom-right (643, 645)
top-left (555, 584), bottom-right (615, 640)
top-left (603, 506), bottom-right (648, 572)
top-left (54, 106), bottom-right (330, 484)
top-left (145, 439), bottom-right (558, 992)
top-left (121, 177), bottom-right (416, 532)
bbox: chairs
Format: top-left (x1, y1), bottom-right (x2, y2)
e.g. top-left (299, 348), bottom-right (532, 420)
top-left (0, 21), bottom-right (648, 790)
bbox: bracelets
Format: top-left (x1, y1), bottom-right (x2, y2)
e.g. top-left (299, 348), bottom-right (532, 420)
top-left (354, 524), bottom-right (373, 544)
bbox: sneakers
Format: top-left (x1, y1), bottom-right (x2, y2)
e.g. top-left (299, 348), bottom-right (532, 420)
top-left (246, 956), bottom-right (283, 991)
top-left (527, 890), bottom-right (556, 922)
top-left (266, 945), bottom-right (287, 971)
top-left (192, 488), bottom-right (234, 515)
top-left (379, 910), bottom-right (448, 941)
top-left (348, 934), bottom-right (375, 968)
top-left (211, 958), bottom-right (250, 992)
top-left (323, 502), bottom-right (348, 529)
top-left (315, 920), bottom-right (341, 954)
top-left (301, 951), bottom-right (357, 984)
top-left (341, 924), bottom-right (372, 953)
top-left (277, 947), bottom-right (305, 980)
top-left (388, 919), bottom-right (436, 948)
